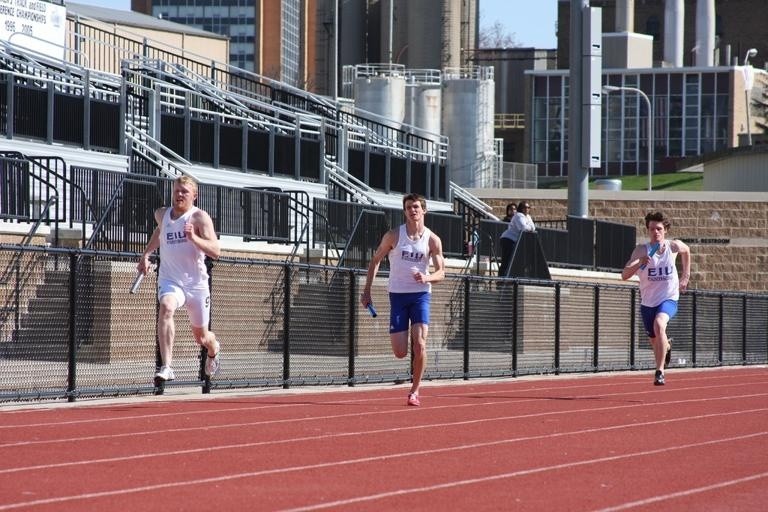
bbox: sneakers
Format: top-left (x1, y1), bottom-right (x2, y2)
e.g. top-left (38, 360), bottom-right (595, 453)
top-left (665, 338), bottom-right (673, 363)
top-left (654, 370), bottom-right (665, 386)
top-left (205, 352), bottom-right (219, 376)
top-left (155, 366), bottom-right (176, 381)
top-left (408, 393), bottom-right (419, 405)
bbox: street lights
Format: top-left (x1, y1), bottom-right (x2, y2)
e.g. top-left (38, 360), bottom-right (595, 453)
top-left (601, 85), bottom-right (652, 190)
top-left (742, 48), bottom-right (758, 145)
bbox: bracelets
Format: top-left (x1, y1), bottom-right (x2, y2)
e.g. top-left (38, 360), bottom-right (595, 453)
top-left (681, 272), bottom-right (690, 283)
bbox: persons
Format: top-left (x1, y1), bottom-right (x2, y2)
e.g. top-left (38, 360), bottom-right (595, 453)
top-left (359, 193), bottom-right (445, 407)
top-left (135, 175), bottom-right (221, 383)
top-left (499, 201), bottom-right (517, 235)
top-left (493, 200), bottom-right (535, 291)
top-left (621, 210), bottom-right (692, 385)
